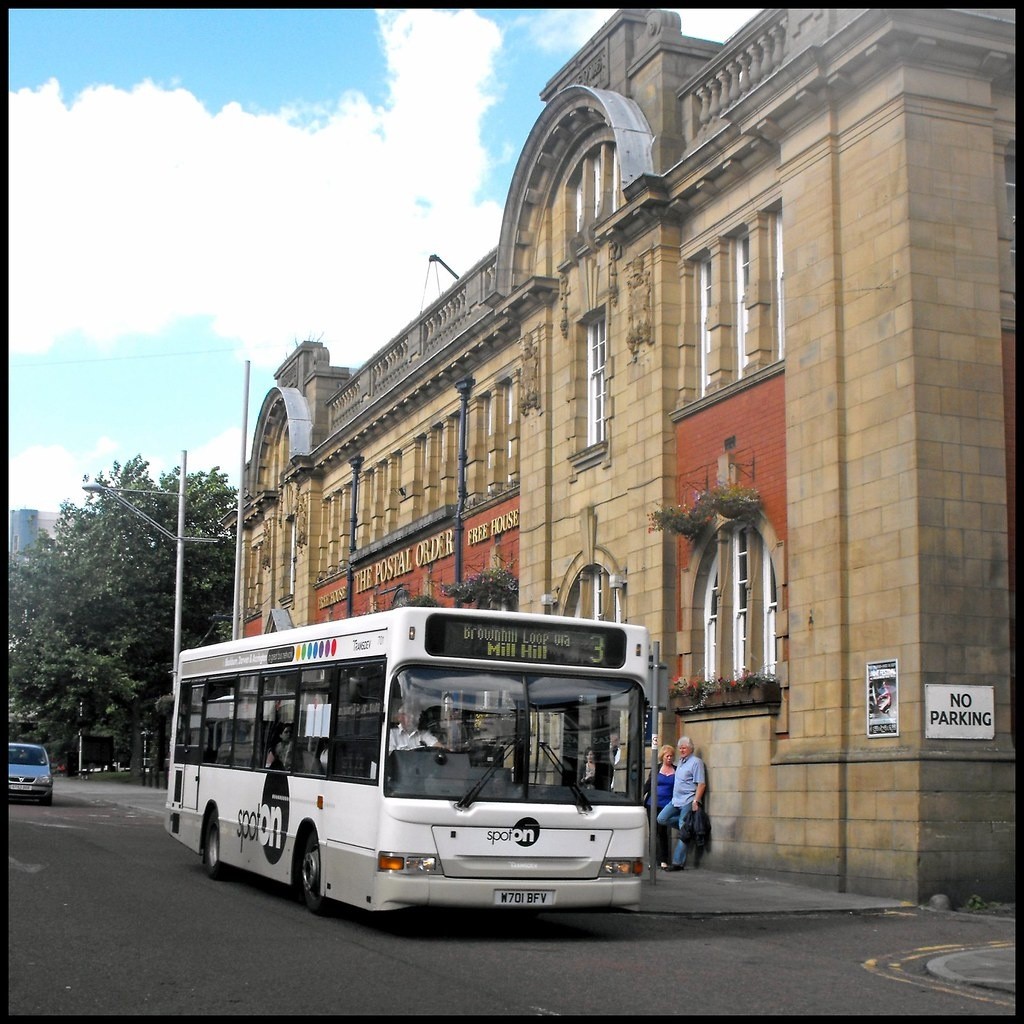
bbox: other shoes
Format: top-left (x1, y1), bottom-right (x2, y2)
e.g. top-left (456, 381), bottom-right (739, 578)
top-left (665, 864), bottom-right (684, 872)
top-left (661, 862), bottom-right (668, 870)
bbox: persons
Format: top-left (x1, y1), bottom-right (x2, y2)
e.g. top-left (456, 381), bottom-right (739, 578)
top-left (643, 745), bottom-right (679, 870)
top-left (389, 703), bottom-right (444, 756)
top-left (265, 726), bottom-right (292, 771)
top-left (656, 736), bottom-right (706, 872)
top-left (579, 732), bottom-right (623, 792)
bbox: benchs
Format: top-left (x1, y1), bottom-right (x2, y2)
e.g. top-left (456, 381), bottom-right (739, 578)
top-left (76, 764), bottom-right (96, 779)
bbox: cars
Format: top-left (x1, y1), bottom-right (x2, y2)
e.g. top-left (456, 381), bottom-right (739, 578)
top-left (8, 743), bottom-right (54, 806)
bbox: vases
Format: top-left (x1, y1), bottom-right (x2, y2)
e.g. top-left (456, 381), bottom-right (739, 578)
top-left (461, 596), bottom-right (476, 604)
top-left (710, 503), bottom-right (745, 519)
top-left (669, 684), bottom-right (777, 715)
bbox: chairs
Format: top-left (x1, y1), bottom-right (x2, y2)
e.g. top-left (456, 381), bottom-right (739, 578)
top-left (326, 734), bottom-right (372, 778)
top-left (204, 719), bottom-right (286, 769)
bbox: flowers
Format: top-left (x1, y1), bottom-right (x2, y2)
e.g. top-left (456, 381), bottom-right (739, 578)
top-left (647, 482), bottom-right (762, 541)
top-left (390, 593), bottom-right (442, 609)
top-left (429, 560), bottom-right (517, 608)
top-left (667, 657), bottom-right (779, 711)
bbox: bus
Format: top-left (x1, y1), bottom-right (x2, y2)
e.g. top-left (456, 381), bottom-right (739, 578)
top-left (164, 605), bottom-right (652, 915)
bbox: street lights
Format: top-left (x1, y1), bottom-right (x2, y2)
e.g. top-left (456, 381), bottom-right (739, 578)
top-left (81, 449), bottom-right (189, 700)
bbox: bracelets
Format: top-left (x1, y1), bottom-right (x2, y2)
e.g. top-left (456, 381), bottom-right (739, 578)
top-left (694, 800), bottom-right (699, 803)
